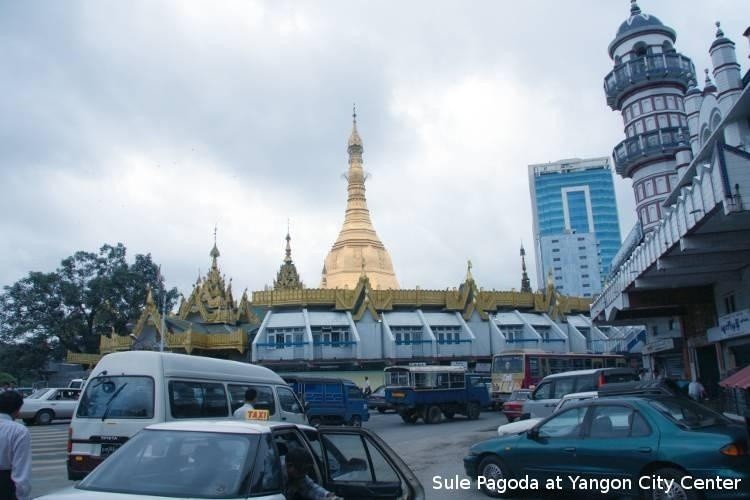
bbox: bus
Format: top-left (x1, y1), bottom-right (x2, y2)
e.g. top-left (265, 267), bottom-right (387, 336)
top-left (491, 347), bottom-right (631, 411)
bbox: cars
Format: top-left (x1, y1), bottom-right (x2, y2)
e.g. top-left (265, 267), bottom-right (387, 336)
top-left (463, 393), bottom-right (749, 500)
top-left (502, 389), bottom-right (535, 422)
top-left (0, 378), bottom-right (87, 425)
top-left (367, 383), bottom-right (393, 410)
top-left (32, 415), bottom-right (426, 500)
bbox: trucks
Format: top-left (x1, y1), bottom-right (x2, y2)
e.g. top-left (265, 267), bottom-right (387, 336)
top-left (281, 375), bottom-right (371, 428)
top-left (385, 362), bottom-right (489, 424)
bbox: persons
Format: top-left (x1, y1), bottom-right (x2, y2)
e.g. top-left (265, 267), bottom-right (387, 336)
top-left (233, 388), bottom-right (258, 417)
top-left (362, 376), bottom-right (370, 395)
top-left (262, 447), bottom-right (345, 500)
top-left (688, 376), bottom-right (707, 404)
top-left (0, 381), bottom-right (38, 398)
top-left (638, 365), bottom-right (664, 380)
top-left (0, 389), bottom-right (32, 500)
top-left (73, 392), bottom-right (80, 399)
top-left (502, 362), bottom-right (512, 373)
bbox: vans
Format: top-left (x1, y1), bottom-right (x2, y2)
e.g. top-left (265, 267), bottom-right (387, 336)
top-left (67, 350), bottom-right (310, 481)
top-left (521, 367), bottom-right (642, 418)
